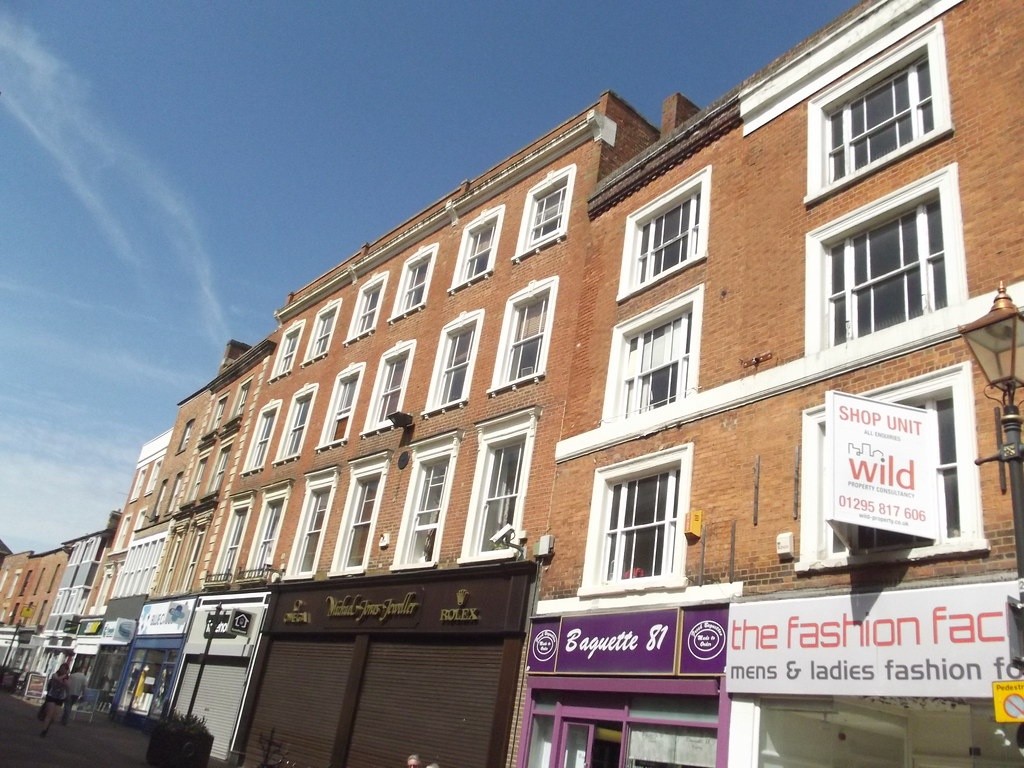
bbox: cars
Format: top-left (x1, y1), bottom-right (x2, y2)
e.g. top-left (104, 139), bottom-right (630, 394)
top-left (0, 664), bottom-right (22, 694)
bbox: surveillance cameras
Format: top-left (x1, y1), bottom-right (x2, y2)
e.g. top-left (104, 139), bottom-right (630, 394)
top-left (489, 524), bottom-right (515, 547)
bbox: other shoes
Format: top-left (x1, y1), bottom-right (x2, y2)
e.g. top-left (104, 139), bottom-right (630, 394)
top-left (41, 729), bottom-right (47, 736)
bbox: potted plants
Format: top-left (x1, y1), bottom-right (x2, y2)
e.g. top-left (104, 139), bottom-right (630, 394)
top-left (146, 710), bottom-right (214, 768)
top-left (0, 666), bottom-right (21, 690)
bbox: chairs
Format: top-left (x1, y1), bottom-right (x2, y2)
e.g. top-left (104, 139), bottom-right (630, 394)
top-left (72, 686), bottom-right (100, 723)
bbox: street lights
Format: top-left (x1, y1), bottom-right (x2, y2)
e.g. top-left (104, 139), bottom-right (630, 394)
top-left (955, 280), bottom-right (1024, 755)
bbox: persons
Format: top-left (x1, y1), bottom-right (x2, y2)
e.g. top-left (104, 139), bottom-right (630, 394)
top-left (407, 754), bottom-right (420, 768)
top-left (426, 763), bottom-right (439, 768)
top-left (58, 666), bottom-right (87, 727)
top-left (39, 664), bottom-right (72, 737)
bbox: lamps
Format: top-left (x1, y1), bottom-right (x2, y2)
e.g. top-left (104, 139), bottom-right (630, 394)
top-left (386, 411), bottom-right (414, 432)
